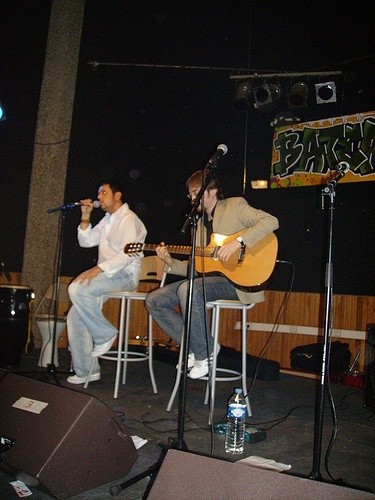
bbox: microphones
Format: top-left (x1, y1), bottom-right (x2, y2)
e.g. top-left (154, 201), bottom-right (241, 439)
top-left (206, 143), bottom-right (228, 169)
top-left (72, 200), bottom-right (100, 208)
top-left (335, 162), bottom-right (351, 173)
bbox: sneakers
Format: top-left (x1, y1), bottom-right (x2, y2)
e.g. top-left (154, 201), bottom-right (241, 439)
top-left (90, 329), bottom-right (119, 357)
top-left (66, 371), bottom-right (101, 384)
top-left (190, 342), bottom-right (222, 379)
top-left (176, 353), bottom-right (196, 370)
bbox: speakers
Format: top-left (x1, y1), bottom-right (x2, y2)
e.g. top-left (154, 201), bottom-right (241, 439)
top-left (362, 323), bottom-right (375, 412)
top-left (0, 286), bottom-right (33, 370)
top-left (0, 373), bottom-right (140, 500)
top-left (142, 446), bottom-right (374, 500)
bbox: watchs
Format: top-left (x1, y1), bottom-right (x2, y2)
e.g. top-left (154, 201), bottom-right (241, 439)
top-left (235, 236), bottom-right (244, 246)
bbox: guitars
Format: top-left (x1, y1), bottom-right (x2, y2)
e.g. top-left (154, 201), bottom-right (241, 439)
top-left (125, 227), bottom-right (279, 292)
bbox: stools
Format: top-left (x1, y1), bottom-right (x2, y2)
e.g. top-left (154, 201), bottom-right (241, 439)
top-left (165, 300), bottom-right (255, 425)
top-left (84, 256), bottom-right (166, 399)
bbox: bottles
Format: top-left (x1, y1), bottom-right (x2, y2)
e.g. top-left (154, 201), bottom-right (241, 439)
top-left (225, 388), bottom-right (246, 454)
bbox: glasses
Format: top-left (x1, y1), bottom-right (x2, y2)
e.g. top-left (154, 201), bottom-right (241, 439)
top-left (186, 191), bottom-right (199, 198)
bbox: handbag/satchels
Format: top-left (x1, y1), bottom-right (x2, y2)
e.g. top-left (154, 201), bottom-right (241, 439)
top-left (296, 340), bottom-right (351, 373)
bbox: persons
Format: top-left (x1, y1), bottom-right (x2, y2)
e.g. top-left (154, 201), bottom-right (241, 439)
top-left (66, 179), bottom-right (147, 385)
top-left (146, 171), bottom-right (280, 379)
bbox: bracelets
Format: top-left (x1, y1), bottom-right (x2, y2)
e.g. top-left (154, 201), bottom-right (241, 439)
top-left (94, 266), bottom-right (100, 273)
top-left (79, 218), bottom-right (90, 223)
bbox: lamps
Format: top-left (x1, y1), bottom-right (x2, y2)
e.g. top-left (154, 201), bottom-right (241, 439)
top-left (232, 75), bottom-right (337, 110)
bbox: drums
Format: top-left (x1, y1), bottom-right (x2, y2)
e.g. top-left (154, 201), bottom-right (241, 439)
top-left (35, 318), bottom-right (66, 368)
top-left (0, 285), bottom-right (34, 366)
